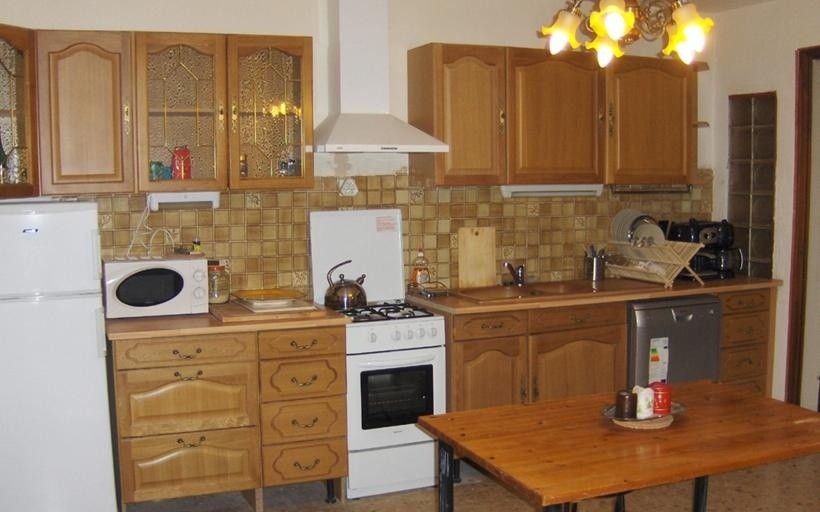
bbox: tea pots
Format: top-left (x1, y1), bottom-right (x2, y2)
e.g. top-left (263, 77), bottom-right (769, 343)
top-left (324, 259), bottom-right (366, 306)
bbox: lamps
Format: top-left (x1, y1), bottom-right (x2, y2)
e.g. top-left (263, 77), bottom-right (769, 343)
top-left (541, 0), bottom-right (714, 68)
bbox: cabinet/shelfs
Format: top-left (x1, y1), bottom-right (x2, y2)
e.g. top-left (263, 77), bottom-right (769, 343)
top-left (0, 23), bottom-right (39, 199)
top-left (716, 287), bottom-right (777, 399)
top-left (607, 55), bottom-right (713, 187)
top-left (34, 28), bottom-right (137, 195)
top-left (111, 334), bottom-right (263, 511)
top-left (444, 302), bottom-right (628, 481)
top-left (408, 42), bottom-right (605, 185)
top-left (257, 327), bottom-right (347, 505)
top-left (134, 27), bottom-right (312, 196)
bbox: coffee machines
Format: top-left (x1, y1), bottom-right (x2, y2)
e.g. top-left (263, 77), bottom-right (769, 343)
top-left (670, 217), bottom-right (744, 280)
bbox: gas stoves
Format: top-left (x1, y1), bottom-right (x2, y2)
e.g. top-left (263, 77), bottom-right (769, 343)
top-left (344, 299), bottom-right (445, 354)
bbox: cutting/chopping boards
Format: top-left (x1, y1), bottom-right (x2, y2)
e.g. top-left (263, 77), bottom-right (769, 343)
top-left (209, 299), bottom-right (325, 324)
top-left (458, 227), bottom-right (498, 290)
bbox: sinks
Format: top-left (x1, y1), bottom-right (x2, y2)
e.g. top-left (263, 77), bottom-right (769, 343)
top-left (447, 284), bottom-right (567, 306)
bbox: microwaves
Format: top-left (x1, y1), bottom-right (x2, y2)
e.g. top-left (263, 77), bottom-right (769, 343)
top-left (104, 258), bottom-right (209, 319)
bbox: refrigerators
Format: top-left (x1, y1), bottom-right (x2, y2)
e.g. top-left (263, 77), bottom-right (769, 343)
top-left (0, 202), bottom-right (117, 512)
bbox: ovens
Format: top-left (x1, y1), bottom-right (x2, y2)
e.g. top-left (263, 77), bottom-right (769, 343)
top-left (346, 346), bottom-right (448, 500)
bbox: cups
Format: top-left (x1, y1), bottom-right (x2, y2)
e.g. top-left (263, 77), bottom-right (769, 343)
top-left (151, 159), bottom-right (171, 180)
top-left (651, 381), bottom-right (670, 414)
top-left (585, 255), bottom-right (603, 280)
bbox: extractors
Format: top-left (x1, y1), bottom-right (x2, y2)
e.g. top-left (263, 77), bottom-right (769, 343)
top-left (311, 1), bottom-right (449, 152)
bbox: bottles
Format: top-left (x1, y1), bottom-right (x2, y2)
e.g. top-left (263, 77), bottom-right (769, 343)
top-left (413, 248), bottom-right (431, 285)
top-left (208, 265), bottom-right (230, 303)
top-left (172, 144), bottom-right (192, 180)
top-left (279, 158), bottom-right (297, 176)
top-left (616, 384), bottom-right (653, 420)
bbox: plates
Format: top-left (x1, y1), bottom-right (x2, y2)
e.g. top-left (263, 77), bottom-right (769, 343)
top-left (610, 209), bottom-right (665, 246)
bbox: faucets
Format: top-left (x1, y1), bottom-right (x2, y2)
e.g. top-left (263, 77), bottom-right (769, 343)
top-left (503, 261), bottom-right (525, 285)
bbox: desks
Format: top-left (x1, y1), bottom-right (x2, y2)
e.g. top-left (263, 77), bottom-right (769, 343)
top-left (417, 380), bottom-right (819, 511)
